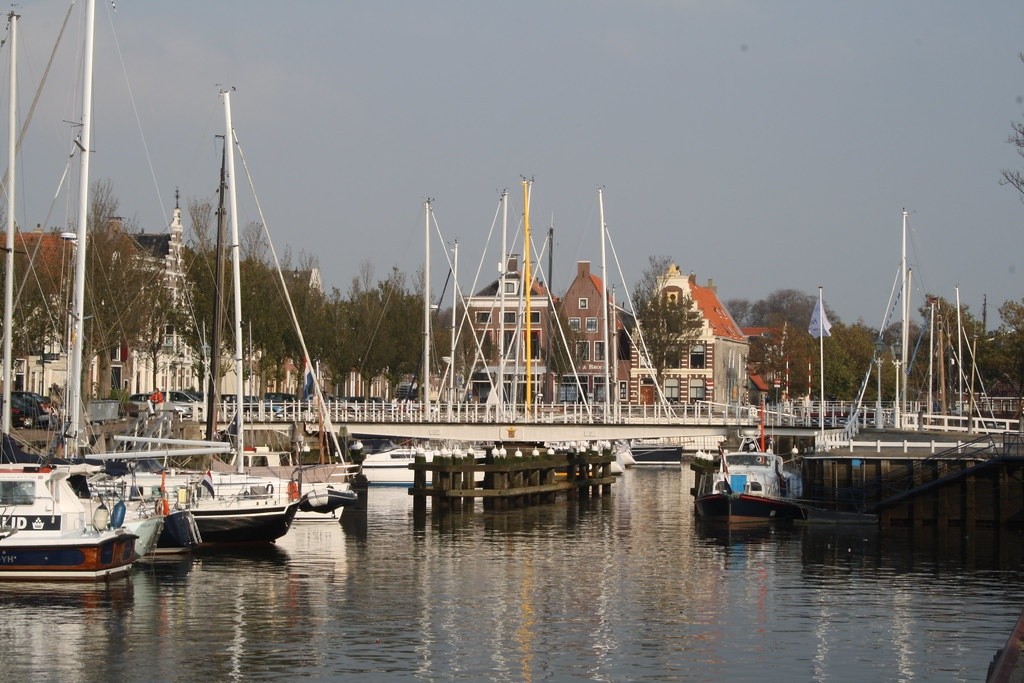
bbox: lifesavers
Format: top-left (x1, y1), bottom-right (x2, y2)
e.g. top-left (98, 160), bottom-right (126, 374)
top-left (288, 481), bottom-right (298, 502)
top-left (155, 498), bottom-right (169, 517)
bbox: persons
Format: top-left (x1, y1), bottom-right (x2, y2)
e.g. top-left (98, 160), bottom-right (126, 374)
top-left (150, 388), bottom-right (163, 411)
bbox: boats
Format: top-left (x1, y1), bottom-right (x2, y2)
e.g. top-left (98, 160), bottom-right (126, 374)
top-left (688, 393), bottom-right (807, 542)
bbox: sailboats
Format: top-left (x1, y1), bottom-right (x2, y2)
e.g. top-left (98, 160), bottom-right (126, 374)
top-left (1, 0), bottom-right (685, 582)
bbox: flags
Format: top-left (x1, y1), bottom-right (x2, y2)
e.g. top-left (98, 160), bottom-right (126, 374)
top-left (304, 351), bottom-right (313, 400)
top-left (808, 298), bottom-right (832, 339)
